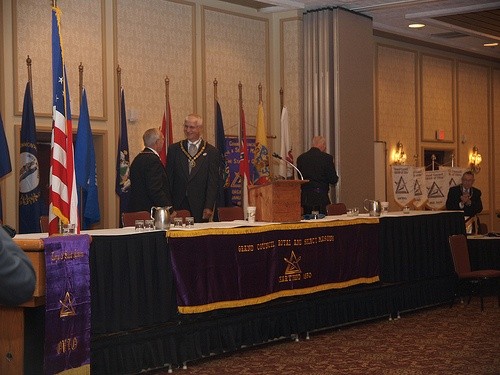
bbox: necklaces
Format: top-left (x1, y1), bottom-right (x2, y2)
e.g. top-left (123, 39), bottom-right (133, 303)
top-left (181, 141), bottom-right (206, 166)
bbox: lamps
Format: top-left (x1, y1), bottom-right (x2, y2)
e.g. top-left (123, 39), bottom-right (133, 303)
top-left (394, 140), bottom-right (408, 163)
top-left (469, 145), bottom-right (482, 171)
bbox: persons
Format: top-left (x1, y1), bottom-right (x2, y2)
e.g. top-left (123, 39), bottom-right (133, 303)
top-left (446, 171), bottom-right (484, 235)
top-left (166, 114), bottom-right (219, 222)
top-left (129, 128), bottom-right (178, 224)
top-left (0, 226), bottom-right (37, 307)
top-left (297, 136), bottom-right (340, 215)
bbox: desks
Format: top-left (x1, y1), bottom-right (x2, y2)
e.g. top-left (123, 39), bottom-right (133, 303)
top-left (16, 209), bottom-right (469, 375)
top-left (467, 235), bottom-right (500, 270)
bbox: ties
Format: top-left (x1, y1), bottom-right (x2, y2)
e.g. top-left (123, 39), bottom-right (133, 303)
top-left (189, 144), bottom-right (197, 174)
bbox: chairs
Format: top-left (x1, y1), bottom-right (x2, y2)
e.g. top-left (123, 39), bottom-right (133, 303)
top-left (447, 234), bottom-right (500, 310)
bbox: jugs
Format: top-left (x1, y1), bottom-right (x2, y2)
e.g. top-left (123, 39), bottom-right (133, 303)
top-left (150, 206), bottom-right (173, 230)
top-left (363, 199), bottom-right (381, 217)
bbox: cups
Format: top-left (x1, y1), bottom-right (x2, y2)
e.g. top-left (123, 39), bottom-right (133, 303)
top-left (67, 224), bottom-right (75, 235)
top-left (403, 205), bottom-right (410, 214)
top-left (63, 228), bottom-right (69, 236)
top-left (347, 208), bottom-right (353, 217)
top-left (247, 206), bottom-right (256, 224)
top-left (135, 219), bottom-right (145, 232)
top-left (354, 208), bottom-right (360, 216)
top-left (381, 202), bottom-right (389, 215)
top-left (312, 211), bottom-right (319, 221)
top-left (185, 216), bottom-right (194, 229)
top-left (145, 220), bottom-right (154, 231)
top-left (173, 218), bottom-right (182, 230)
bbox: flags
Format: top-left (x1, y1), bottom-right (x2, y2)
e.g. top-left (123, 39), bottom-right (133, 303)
top-left (238, 102), bottom-right (252, 220)
top-left (279, 104), bottom-right (296, 179)
top-left (115, 87), bottom-right (131, 227)
top-left (0, 7), bottom-right (101, 236)
top-left (210, 101), bottom-right (233, 221)
top-left (252, 101), bottom-right (271, 186)
